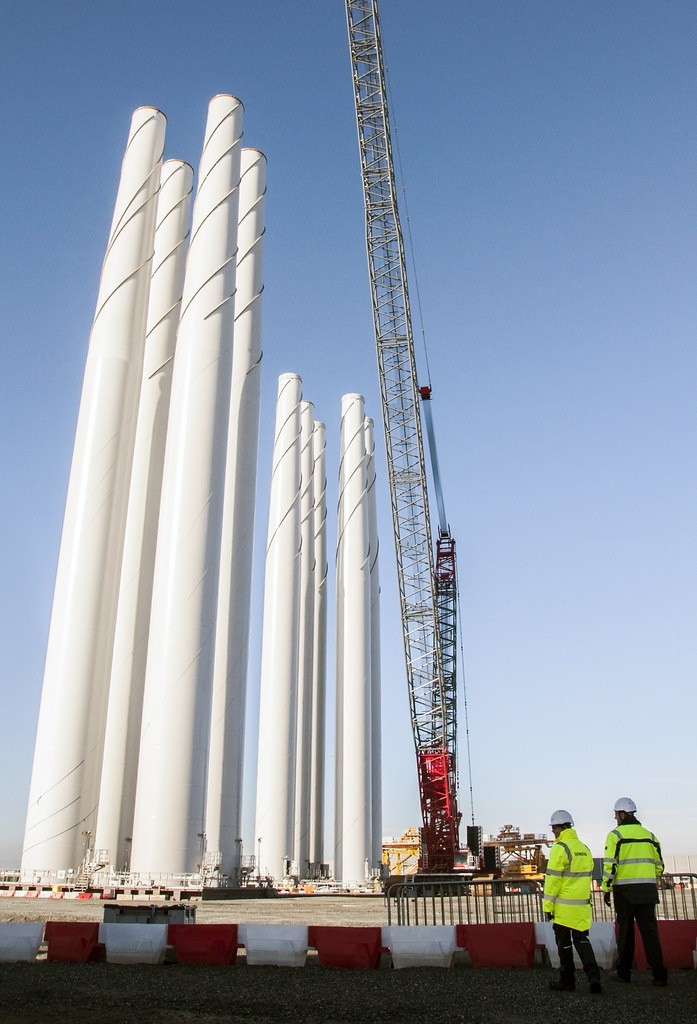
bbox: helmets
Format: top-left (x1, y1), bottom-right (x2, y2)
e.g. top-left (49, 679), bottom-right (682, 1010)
top-left (549, 810), bottom-right (574, 826)
top-left (613, 797), bottom-right (637, 812)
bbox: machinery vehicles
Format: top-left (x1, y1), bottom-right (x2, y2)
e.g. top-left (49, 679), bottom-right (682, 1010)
top-left (337, 0), bottom-right (558, 896)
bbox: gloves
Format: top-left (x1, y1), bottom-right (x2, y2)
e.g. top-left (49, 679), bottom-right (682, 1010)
top-left (546, 912), bottom-right (554, 920)
top-left (604, 892), bottom-right (611, 906)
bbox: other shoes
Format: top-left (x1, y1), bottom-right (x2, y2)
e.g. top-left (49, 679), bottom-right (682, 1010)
top-left (610, 974), bottom-right (629, 983)
top-left (589, 980), bottom-right (602, 993)
top-left (651, 976), bottom-right (668, 986)
top-left (549, 981), bottom-right (576, 991)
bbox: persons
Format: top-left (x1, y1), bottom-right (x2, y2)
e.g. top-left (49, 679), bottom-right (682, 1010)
top-left (541, 811), bottom-right (602, 994)
top-left (601, 797), bottom-right (668, 988)
